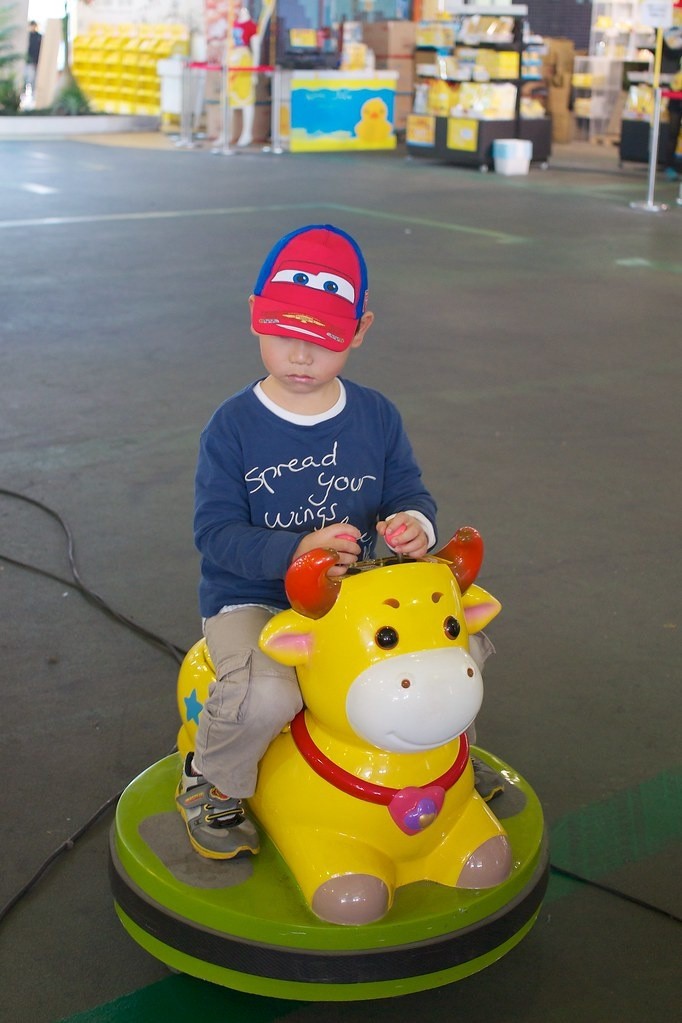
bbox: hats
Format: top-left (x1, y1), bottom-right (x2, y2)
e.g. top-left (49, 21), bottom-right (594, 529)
top-left (251, 223), bottom-right (370, 352)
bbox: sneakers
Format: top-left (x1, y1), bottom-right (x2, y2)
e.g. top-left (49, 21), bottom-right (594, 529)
top-left (174, 750), bottom-right (261, 859)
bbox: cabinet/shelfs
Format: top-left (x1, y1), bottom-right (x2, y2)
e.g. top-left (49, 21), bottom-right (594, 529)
top-left (72, 22), bottom-right (189, 114)
top-left (407, 12), bottom-right (553, 172)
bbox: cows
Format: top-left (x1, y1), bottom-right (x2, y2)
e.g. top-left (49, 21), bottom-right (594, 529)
top-left (177, 526), bottom-right (509, 925)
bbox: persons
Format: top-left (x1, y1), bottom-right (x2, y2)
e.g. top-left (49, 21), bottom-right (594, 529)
top-left (216, 7), bottom-right (260, 147)
top-left (23, 21), bottom-right (41, 91)
top-left (172, 225), bottom-right (508, 861)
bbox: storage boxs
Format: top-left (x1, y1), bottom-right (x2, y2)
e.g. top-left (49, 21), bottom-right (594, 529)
top-left (492, 138), bottom-right (534, 176)
top-left (361, 22), bottom-right (414, 131)
top-left (476, 48), bottom-right (520, 81)
top-left (415, 21), bottom-right (456, 51)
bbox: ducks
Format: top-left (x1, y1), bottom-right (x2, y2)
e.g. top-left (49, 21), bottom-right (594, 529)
top-left (354, 96), bottom-right (393, 138)
